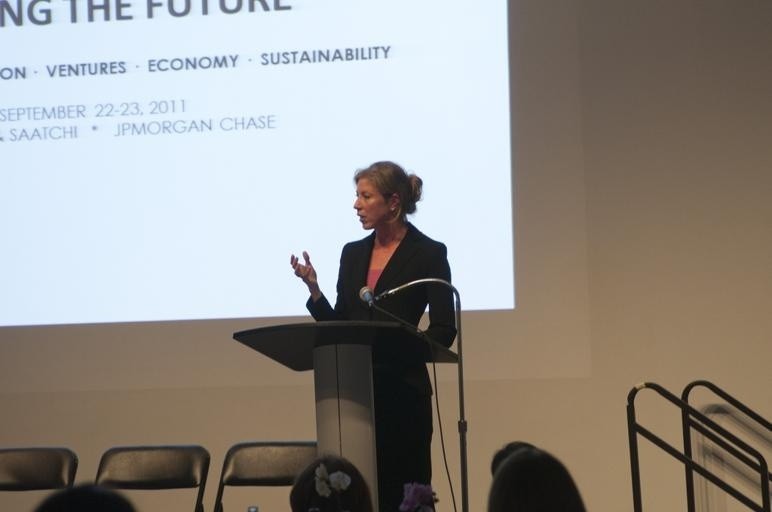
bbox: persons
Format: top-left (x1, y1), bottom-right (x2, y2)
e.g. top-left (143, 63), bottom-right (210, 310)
top-left (490, 439), bottom-right (534, 476)
top-left (290, 452), bottom-right (374, 511)
top-left (487, 445), bottom-right (586, 511)
top-left (291, 157), bottom-right (459, 511)
top-left (31, 483), bottom-right (139, 511)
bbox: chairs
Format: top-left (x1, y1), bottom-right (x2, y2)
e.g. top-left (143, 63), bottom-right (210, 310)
top-left (0, 448), bottom-right (78, 490)
top-left (96, 445), bottom-right (210, 512)
top-left (213, 441), bottom-right (317, 512)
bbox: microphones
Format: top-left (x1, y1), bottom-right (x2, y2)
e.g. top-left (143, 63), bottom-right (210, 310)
top-left (359, 287), bottom-right (376, 309)
